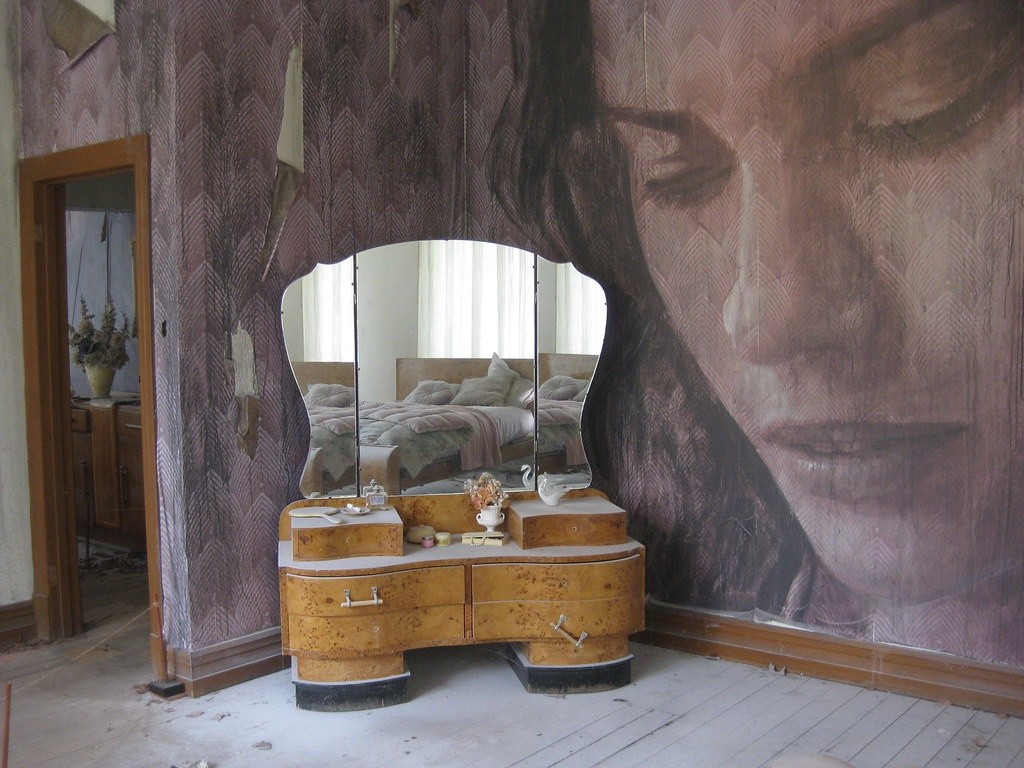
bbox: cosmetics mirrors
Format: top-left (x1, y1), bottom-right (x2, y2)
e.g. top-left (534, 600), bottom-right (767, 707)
top-left (288, 506), bottom-right (343, 524)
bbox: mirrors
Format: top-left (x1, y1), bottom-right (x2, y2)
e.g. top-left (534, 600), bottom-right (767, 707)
top-left (281, 239), bottom-right (608, 500)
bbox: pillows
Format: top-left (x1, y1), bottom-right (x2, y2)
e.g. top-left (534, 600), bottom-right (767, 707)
top-left (303, 387), bottom-right (354, 409)
top-left (450, 375), bottom-right (515, 405)
top-left (413, 382), bottom-right (452, 404)
top-left (488, 353), bottom-right (535, 408)
top-left (402, 381), bottom-right (461, 405)
top-left (306, 384), bottom-right (350, 407)
top-left (539, 375), bottom-right (577, 399)
top-left (570, 379), bottom-right (591, 401)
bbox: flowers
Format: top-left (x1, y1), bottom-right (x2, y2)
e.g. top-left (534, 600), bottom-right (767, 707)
top-left (462, 472), bottom-right (496, 492)
top-left (470, 480), bottom-right (509, 509)
top-left (69, 294), bottom-right (135, 367)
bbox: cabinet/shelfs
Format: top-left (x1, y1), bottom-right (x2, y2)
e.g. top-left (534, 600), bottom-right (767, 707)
top-left (279, 488), bottom-right (644, 712)
top-left (72, 391), bottom-right (146, 554)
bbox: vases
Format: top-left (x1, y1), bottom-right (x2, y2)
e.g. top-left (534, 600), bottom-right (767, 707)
top-left (476, 506), bottom-right (506, 532)
top-left (83, 362), bottom-right (117, 398)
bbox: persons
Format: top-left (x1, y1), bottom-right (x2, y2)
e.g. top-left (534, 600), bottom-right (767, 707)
top-left (479, 1), bottom-right (1024, 691)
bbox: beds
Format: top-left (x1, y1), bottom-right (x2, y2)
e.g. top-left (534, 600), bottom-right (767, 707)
top-left (293, 363), bottom-right (354, 493)
top-left (357, 358), bottom-right (533, 495)
top-left (539, 353), bottom-right (599, 466)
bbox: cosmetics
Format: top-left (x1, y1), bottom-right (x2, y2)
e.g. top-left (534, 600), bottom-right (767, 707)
top-left (420, 535), bottom-right (436, 549)
top-left (405, 521), bottom-right (439, 543)
top-left (434, 531), bottom-right (452, 546)
top-left (346, 503), bottom-right (364, 512)
top-left (364, 486), bottom-right (390, 513)
